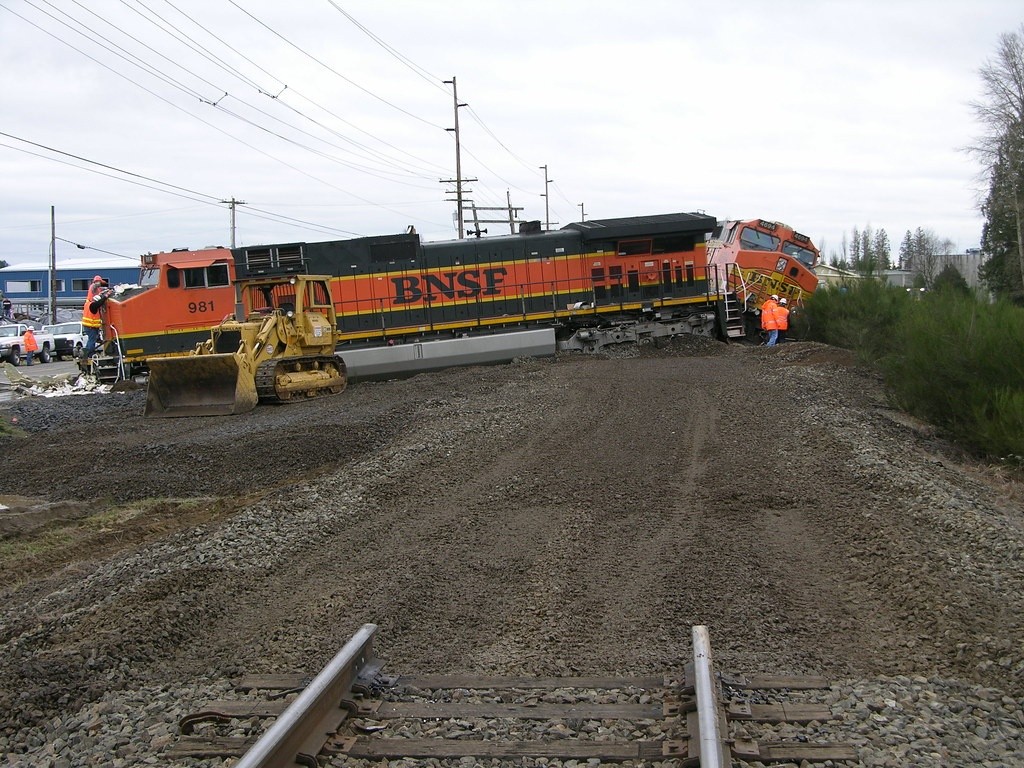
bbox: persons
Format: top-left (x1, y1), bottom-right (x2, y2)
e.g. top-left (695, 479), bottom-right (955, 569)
top-left (24, 325), bottom-right (39, 366)
top-left (81, 274), bottom-right (115, 359)
top-left (0, 298), bottom-right (12, 317)
top-left (759, 294), bottom-right (791, 347)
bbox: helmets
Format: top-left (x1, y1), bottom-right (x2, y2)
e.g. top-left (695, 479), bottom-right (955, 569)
top-left (93, 295), bottom-right (101, 302)
top-left (28, 326), bottom-right (34, 331)
top-left (93, 275), bottom-right (102, 282)
top-left (779, 298), bottom-right (786, 304)
top-left (771, 295), bottom-right (778, 301)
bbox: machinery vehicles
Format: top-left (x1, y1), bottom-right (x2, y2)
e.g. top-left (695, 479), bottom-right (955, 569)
top-left (143, 274), bottom-right (348, 419)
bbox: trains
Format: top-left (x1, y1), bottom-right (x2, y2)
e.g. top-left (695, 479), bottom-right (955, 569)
top-left (92, 211), bottom-right (720, 387)
top-left (703, 217), bottom-right (821, 330)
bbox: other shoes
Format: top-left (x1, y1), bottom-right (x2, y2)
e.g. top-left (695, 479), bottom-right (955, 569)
top-left (96, 338), bottom-right (102, 343)
top-left (89, 356), bottom-right (99, 358)
top-left (27, 365), bottom-right (34, 366)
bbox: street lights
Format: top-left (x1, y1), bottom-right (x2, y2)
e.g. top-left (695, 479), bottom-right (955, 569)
top-left (48, 238), bottom-right (85, 325)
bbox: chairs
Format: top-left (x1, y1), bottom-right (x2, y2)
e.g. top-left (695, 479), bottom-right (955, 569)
top-left (278, 303), bottom-right (294, 316)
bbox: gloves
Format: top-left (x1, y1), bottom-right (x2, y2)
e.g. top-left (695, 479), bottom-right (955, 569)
top-left (104, 290), bottom-right (113, 298)
top-left (102, 281), bottom-right (106, 285)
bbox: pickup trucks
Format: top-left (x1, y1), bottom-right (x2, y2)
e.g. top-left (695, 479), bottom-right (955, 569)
top-left (0, 323), bottom-right (56, 366)
top-left (41, 321), bottom-right (105, 362)
